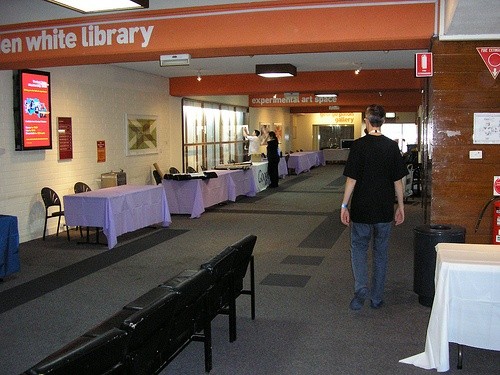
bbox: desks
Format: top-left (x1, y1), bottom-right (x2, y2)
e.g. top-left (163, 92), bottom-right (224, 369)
top-left (163, 151), bottom-right (320, 216)
top-left (63, 184), bottom-right (163, 247)
top-left (437, 243), bottom-right (500, 369)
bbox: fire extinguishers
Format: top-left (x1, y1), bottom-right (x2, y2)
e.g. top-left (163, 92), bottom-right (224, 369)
top-left (475, 196), bottom-right (500, 245)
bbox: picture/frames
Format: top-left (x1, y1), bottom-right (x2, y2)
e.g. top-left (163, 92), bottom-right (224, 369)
top-left (124, 115), bottom-right (159, 155)
top-left (260, 122), bottom-right (284, 144)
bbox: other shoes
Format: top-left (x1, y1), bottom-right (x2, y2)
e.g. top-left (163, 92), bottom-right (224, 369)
top-left (267, 184), bottom-right (278, 188)
top-left (351, 287), bottom-right (368, 309)
top-left (371, 300), bottom-right (383, 308)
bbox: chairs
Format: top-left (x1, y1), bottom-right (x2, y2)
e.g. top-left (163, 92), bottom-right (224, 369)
top-left (19, 235), bottom-right (259, 375)
top-left (186, 167), bottom-right (196, 172)
top-left (75, 183), bottom-right (90, 194)
top-left (170, 167), bottom-right (179, 173)
top-left (153, 171), bottom-right (162, 184)
top-left (41, 181), bottom-right (82, 239)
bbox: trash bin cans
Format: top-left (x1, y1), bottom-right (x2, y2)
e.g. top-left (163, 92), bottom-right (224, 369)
top-left (413, 224), bottom-right (466, 306)
top-left (101, 172), bottom-right (126, 188)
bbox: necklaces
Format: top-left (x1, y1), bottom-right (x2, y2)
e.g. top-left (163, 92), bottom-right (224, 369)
top-left (369, 130), bottom-right (381, 134)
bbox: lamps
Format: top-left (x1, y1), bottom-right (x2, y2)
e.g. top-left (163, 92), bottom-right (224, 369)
top-left (256, 63), bottom-right (297, 79)
top-left (196, 71), bottom-right (201, 81)
top-left (45, 0), bottom-right (150, 14)
top-left (316, 92), bottom-right (338, 98)
top-left (355, 67), bottom-right (362, 74)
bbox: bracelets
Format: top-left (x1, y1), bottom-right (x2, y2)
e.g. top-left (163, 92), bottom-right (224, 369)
top-left (342, 203), bottom-right (347, 207)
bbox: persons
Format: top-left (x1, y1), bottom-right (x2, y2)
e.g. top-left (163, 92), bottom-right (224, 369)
top-left (340, 106), bottom-right (404, 311)
top-left (261, 132), bottom-right (280, 188)
top-left (242, 127), bottom-right (261, 162)
